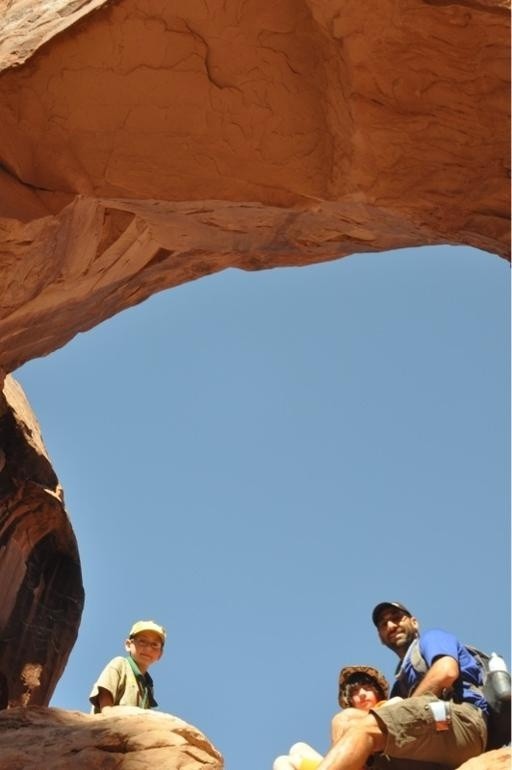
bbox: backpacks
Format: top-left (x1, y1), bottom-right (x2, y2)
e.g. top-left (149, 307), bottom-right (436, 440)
top-left (408, 638), bottom-right (511, 719)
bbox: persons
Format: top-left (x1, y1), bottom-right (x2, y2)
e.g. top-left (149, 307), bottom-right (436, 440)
top-left (318, 601), bottom-right (489, 770)
top-left (89, 619), bottom-right (166, 716)
top-left (271, 664), bottom-right (391, 770)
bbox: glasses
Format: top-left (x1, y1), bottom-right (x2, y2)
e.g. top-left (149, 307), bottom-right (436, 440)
top-left (132, 638), bottom-right (162, 650)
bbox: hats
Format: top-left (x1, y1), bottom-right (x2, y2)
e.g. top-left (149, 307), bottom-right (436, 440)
top-left (129, 620), bottom-right (167, 643)
top-left (337, 664), bottom-right (389, 709)
top-left (372, 600), bottom-right (412, 629)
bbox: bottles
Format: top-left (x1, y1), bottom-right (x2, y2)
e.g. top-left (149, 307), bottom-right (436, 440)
top-left (489, 652), bottom-right (510, 698)
